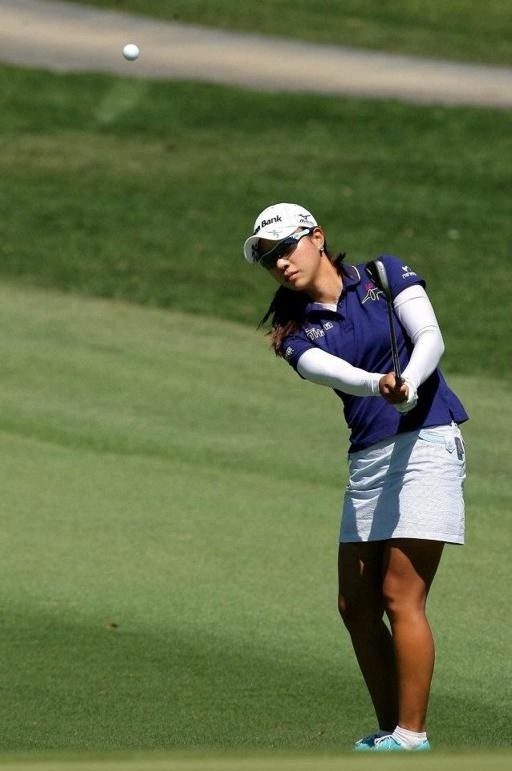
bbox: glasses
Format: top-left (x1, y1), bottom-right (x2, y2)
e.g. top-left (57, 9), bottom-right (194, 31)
top-left (259, 227), bottom-right (313, 271)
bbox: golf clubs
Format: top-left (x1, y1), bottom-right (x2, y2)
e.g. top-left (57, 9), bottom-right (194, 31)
top-left (366, 259), bottom-right (403, 387)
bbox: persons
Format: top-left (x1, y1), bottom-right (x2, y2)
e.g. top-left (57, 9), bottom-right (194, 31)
top-left (242, 201), bottom-right (471, 756)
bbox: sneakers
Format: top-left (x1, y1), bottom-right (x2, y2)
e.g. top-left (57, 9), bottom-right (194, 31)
top-left (355, 731), bottom-right (391, 745)
top-left (352, 737), bottom-right (431, 753)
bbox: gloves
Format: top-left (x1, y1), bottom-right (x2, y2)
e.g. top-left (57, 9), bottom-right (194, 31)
top-left (393, 381), bottom-right (419, 414)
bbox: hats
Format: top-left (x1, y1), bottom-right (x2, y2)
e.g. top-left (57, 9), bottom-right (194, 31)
top-left (243, 201), bottom-right (321, 266)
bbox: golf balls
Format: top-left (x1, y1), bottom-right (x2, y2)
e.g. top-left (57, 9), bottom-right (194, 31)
top-left (123, 44), bottom-right (139, 59)
